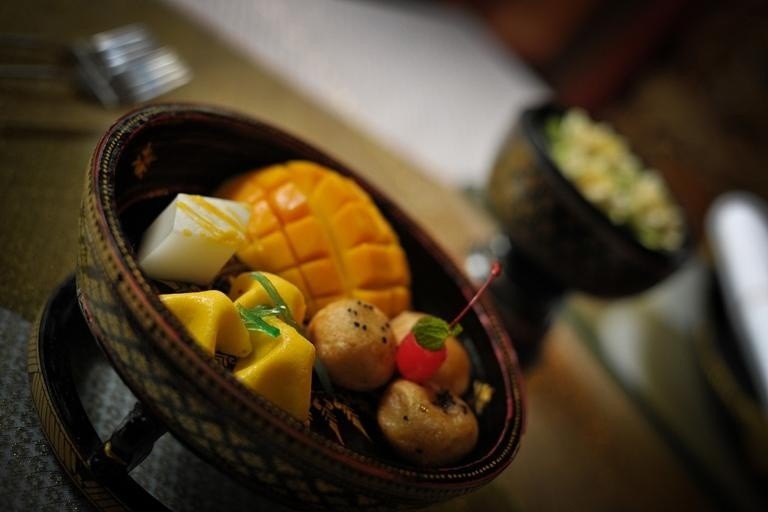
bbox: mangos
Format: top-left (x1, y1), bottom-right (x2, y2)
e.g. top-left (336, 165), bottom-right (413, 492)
top-left (211, 156), bottom-right (411, 323)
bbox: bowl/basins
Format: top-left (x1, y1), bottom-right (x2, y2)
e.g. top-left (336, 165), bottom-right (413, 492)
top-left (485, 100), bottom-right (700, 298)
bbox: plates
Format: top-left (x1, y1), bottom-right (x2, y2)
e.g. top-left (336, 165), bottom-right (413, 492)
top-left (72, 98), bottom-right (530, 503)
